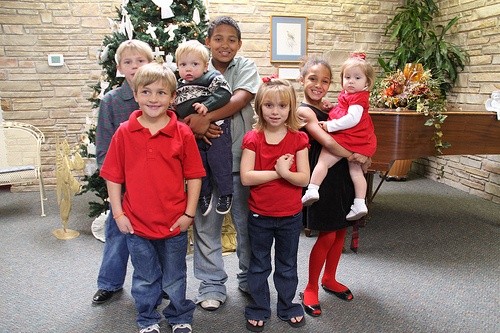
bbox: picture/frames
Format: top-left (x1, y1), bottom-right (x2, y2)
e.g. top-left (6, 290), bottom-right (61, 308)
top-left (270, 15), bottom-right (308, 63)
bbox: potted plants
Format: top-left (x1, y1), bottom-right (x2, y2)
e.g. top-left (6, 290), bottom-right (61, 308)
top-left (372, 0), bottom-right (472, 177)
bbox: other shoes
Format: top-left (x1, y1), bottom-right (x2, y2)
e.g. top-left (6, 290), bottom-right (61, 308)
top-left (345, 203), bottom-right (368, 221)
top-left (200, 300), bottom-right (220, 311)
top-left (301, 191), bottom-right (319, 206)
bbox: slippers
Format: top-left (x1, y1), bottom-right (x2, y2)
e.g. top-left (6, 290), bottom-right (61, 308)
top-left (299, 292), bottom-right (322, 317)
top-left (322, 283), bottom-right (354, 301)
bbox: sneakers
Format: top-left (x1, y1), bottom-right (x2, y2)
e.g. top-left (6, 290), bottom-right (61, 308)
top-left (215, 193), bottom-right (233, 215)
top-left (198, 192), bottom-right (213, 217)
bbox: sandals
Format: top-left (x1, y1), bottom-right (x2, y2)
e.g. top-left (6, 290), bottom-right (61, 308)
top-left (288, 315), bottom-right (306, 328)
top-left (139, 323), bottom-right (160, 333)
top-left (172, 323), bottom-right (193, 333)
top-left (246, 319), bottom-right (264, 332)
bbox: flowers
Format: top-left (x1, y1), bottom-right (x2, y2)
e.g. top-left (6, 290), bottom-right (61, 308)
top-left (370, 58), bottom-right (453, 180)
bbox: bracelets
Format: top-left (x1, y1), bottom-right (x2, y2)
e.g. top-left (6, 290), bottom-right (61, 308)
top-left (113, 212), bottom-right (124, 219)
top-left (184, 213), bottom-right (195, 219)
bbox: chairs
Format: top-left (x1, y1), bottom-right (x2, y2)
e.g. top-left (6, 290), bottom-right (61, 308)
top-left (0, 121), bottom-right (48, 217)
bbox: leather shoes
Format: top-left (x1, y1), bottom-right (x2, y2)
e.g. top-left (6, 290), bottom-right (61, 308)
top-left (162, 292), bottom-right (169, 299)
top-left (93, 287), bottom-right (122, 304)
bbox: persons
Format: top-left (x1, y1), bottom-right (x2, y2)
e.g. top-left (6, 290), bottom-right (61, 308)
top-left (301, 50), bottom-right (377, 220)
top-left (296, 52), bottom-right (372, 317)
top-left (93, 39), bottom-right (169, 304)
top-left (168, 40), bottom-right (234, 216)
top-left (193, 15), bottom-right (263, 308)
top-left (100, 63), bottom-right (207, 333)
top-left (240, 74), bottom-right (310, 333)
